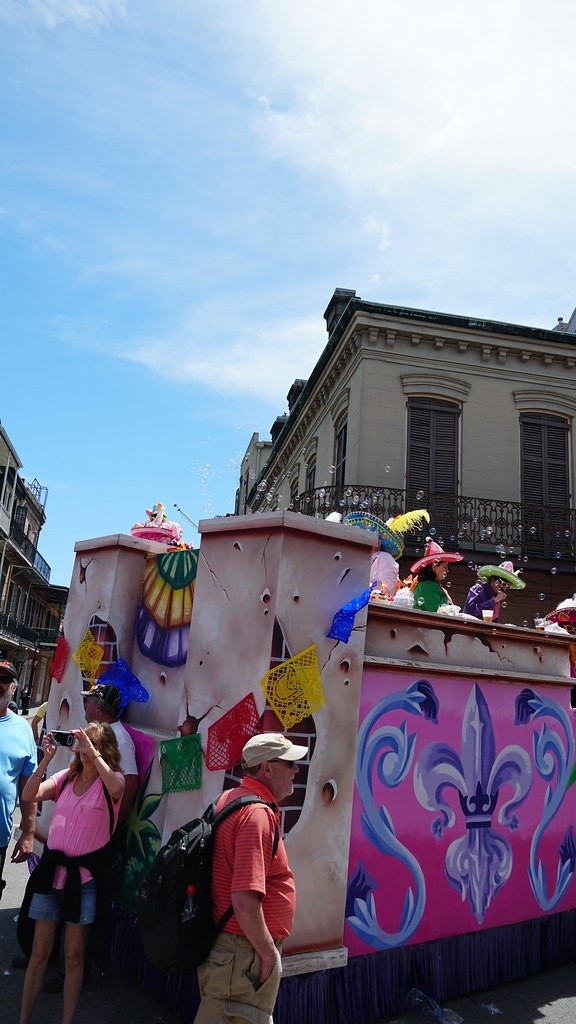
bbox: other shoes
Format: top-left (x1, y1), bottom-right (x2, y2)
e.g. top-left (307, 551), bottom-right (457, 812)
top-left (12, 954), bottom-right (29, 969)
top-left (43, 970), bottom-right (66, 992)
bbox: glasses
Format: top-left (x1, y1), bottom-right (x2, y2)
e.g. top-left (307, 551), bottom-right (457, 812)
top-left (0, 676), bottom-right (15, 684)
top-left (563, 624), bottom-right (576, 633)
top-left (499, 577), bottom-right (512, 590)
top-left (267, 759), bottom-right (293, 769)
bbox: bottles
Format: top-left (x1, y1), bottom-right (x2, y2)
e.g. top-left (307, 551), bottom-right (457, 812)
top-left (181, 885), bottom-right (198, 923)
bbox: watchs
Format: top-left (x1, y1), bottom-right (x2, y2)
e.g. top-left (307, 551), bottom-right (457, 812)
top-left (90, 750), bottom-right (102, 761)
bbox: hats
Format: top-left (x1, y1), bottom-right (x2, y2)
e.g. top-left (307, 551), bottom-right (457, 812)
top-left (409, 541), bottom-right (464, 572)
top-left (342, 508), bottom-right (431, 560)
top-left (543, 599), bottom-right (576, 634)
top-left (241, 733), bottom-right (309, 768)
top-left (0, 659), bottom-right (18, 681)
top-left (79, 683), bottom-right (120, 715)
top-left (477, 561), bottom-right (526, 591)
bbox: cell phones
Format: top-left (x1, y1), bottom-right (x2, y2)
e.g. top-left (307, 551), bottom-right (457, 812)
top-left (49, 729), bottom-right (76, 747)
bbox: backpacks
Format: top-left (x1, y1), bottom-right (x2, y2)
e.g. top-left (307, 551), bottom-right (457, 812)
top-left (134, 789), bottom-right (279, 976)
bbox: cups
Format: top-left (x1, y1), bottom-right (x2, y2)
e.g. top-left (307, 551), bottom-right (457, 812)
top-left (534, 619), bottom-right (545, 631)
top-left (482, 610), bottom-right (494, 623)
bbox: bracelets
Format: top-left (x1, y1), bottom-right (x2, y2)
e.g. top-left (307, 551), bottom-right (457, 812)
top-left (33, 771), bottom-right (45, 780)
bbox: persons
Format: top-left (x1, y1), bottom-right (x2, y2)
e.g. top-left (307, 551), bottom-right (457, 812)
top-left (559, 623), bottom-right (576, 679)
top-left (191, 733), bottom-right (309, 1023)
top-left (19, 721), bottom-right (119, 1024)
top-left (43, 683), bottom-right (141, 1001)
top-left (370, 535), bottom-right (399, 603)
top-left (8, 677), bottom-right (20, 716)
top-left (463, 561), bottom-right (526, 622)
top-left (20, 684), bottom-right (31, 712)
top-left (31, 700), bottom-right (48, 817)
top-left (410, 539), bottom-right (463, 616)
top-left (0, 659), bottom-right (39, 901)
